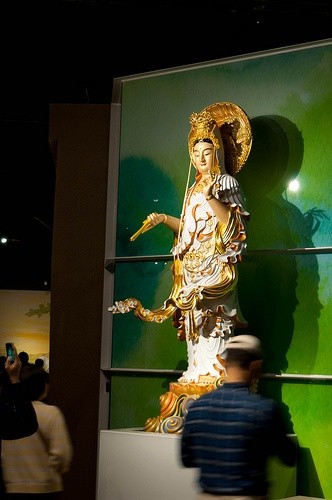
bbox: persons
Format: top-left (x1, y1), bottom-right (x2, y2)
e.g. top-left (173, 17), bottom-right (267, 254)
top-left (0, 343), bottom-right (73, 500)
top-left (179, 336), bottom-right (302, 500)
top-left (107, 102), bottom-right (253, 429)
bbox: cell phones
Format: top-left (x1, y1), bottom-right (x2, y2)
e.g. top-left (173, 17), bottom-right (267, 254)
top-left (6, 342), bottom-right (15, 364)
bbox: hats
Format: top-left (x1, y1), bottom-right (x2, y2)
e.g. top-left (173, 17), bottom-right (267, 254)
top-left (221, 335), bottom-right (261, 354)
